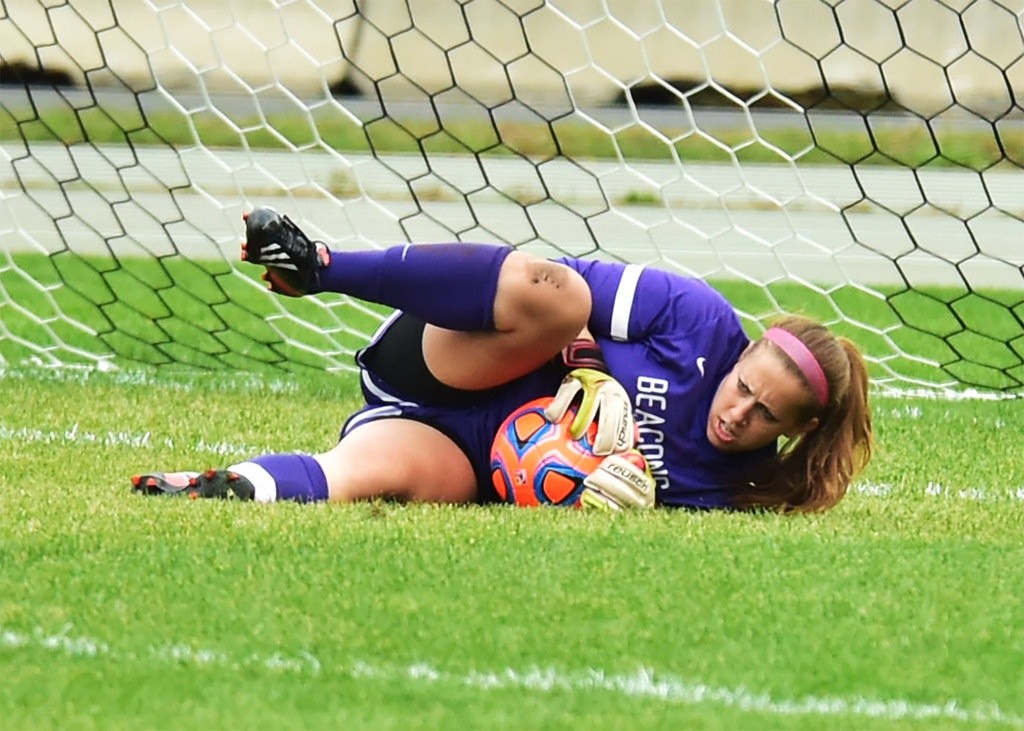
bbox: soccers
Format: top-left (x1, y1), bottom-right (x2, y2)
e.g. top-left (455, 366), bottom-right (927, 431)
top-left (489, 396), bottom-right (606, 507)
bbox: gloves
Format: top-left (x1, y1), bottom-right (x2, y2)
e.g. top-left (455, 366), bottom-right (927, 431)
top-left (543, 337), bottom-right (639, 456)
top-left (580, 450), bottom-right (655, 512)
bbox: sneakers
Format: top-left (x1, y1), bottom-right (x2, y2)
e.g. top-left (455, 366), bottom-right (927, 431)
top-left (130, 468), bottom-right (255, 503)
top-left (240, 207), bottom-right (331, 298)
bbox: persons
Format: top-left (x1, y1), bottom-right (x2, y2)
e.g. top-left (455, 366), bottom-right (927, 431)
top-left (129, 207), bottom-right (874, 512)
